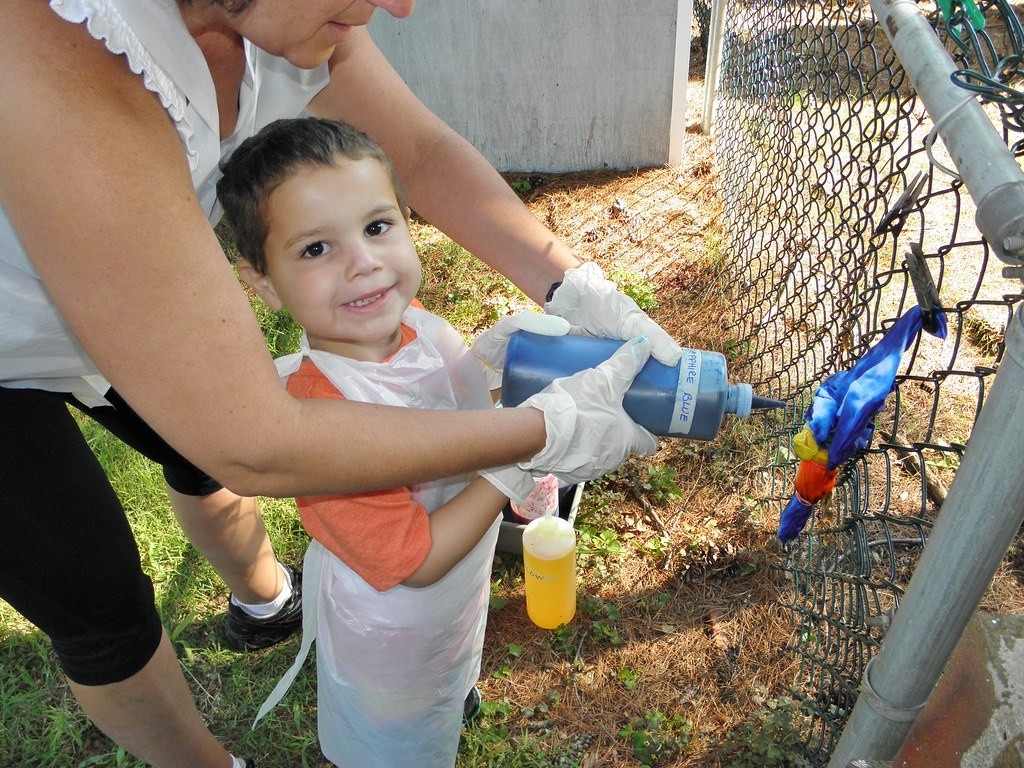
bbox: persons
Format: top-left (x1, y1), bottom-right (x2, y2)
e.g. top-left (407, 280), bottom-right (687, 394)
top-left (0, 0), bottom-right (683, 768)
top-left (210, 115), bottom-right (573, 767)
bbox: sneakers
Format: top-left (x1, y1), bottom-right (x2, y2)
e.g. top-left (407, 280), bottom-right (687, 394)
top-left (220, 562), bottom-right (302, 652)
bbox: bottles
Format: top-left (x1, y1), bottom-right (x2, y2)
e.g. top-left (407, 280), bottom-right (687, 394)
top-left (501, 332), bottom-right (786, 442)
top-left (510, 471), bottom-right (559, 525)
top-left (523, 508), bottom-right (576, 630)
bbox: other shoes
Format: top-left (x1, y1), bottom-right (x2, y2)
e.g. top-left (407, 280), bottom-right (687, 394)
top-left (463, 685), bottom-right (481, 723)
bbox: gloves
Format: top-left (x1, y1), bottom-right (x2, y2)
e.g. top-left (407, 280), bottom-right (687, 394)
top-left (545, 261), bottom-right (681, 368)
top-left (477, 462), bottom-right (549, 504)
top-left (515, 335), bottom-right (660, 484)
top-left (473, 309), bottom-right (570, 390)
